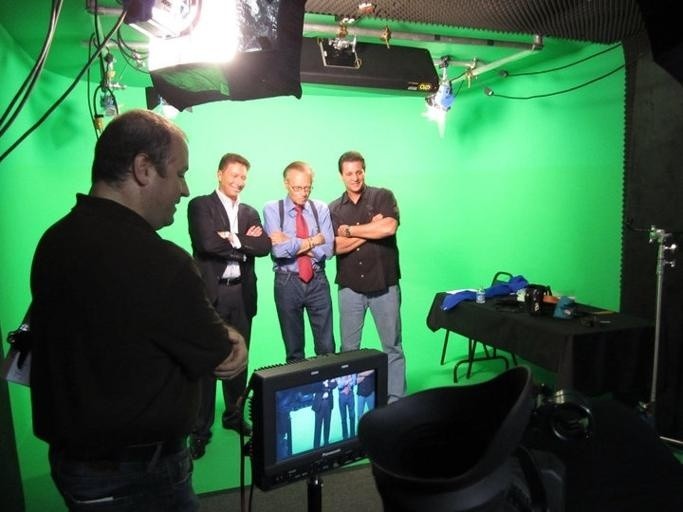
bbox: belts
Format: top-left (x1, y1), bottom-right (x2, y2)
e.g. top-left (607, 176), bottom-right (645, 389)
top-left (64, 441), bottom-right (189, 460)
top-left (219, 278), bottom-right (240, 284)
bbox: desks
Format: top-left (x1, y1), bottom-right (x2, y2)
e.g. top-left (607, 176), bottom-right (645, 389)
top-left (426, 271), bottom-right (655, 397)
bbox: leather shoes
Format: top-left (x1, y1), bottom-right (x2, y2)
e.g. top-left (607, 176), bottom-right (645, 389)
top-left (189, 436), bottom-right (205, 458)
top-left (223, 419), bottom-right (252, 436)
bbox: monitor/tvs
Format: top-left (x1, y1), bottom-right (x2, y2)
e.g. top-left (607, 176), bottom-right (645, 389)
top-left (252, 350), bottom-right (389, 492)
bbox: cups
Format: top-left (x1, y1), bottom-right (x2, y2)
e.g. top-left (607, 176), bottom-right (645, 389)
top-left (543, 295), bottom-right (558, 315)
top-left (474, 289), bottom-right (484, 305)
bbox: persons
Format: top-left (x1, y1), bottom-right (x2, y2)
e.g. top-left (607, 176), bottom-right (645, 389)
top-left (355, 369), bottom-right (375, 421)
top-left (29, 109), bottom-right (249, 512)
top-left (335, 373), bottom-right (357, 440)
top-left (262, 160), bottom-right (335, 365)
top-left (327, 150), bottom-right (407, 406)
top-left (311, 378), bottom-right (337, 451)
top-left (185, 153), bottom-right (272, 460)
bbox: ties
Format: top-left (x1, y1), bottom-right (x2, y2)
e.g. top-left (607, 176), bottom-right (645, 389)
top-left (295, 206), bottom-right (314, 283)
top-left (344, 376), bottom-right (349, 395)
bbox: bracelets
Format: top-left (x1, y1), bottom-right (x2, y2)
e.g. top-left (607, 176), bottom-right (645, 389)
top-left (306, 236), bottom-right (315, 250)
top-left (345, 381), bottom-right (349, 385)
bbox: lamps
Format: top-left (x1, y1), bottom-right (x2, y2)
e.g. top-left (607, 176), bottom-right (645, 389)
top-left (86, 0), bottom-right (305, 116)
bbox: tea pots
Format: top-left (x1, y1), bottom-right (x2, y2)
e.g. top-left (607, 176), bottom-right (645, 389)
top-left (523, 283), bottom-right (552, 317)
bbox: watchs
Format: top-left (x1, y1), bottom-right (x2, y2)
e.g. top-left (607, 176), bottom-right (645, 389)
top-left (345, 224), bottom-right (351, 239)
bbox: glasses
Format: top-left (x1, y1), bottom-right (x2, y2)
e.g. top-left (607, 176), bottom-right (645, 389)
top-left (291, 184), bottom-right (311, 192)
